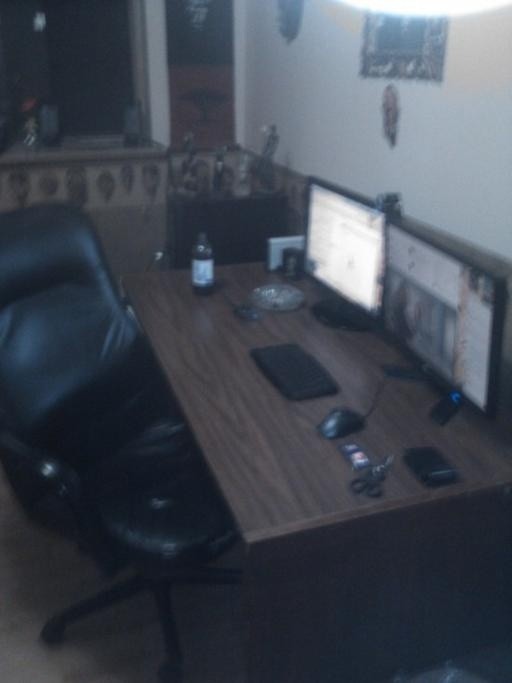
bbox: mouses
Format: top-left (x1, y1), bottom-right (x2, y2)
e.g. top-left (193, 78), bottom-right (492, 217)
top-left (316, 405), bottom-right (366, 438)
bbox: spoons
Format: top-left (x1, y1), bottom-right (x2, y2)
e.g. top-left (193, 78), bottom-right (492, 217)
top-left (223, 291), bottom-right (258, 321)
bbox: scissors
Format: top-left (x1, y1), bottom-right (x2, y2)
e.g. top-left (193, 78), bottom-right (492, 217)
top-left (350, 452), bottom-right (394, 497)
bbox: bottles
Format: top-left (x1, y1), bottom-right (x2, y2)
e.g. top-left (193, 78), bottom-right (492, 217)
top-left (191, 230), bottom-right (215, 294)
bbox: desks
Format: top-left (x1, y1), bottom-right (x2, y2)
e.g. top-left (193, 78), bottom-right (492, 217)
top-left (119, 260), bottom-right (511, 683)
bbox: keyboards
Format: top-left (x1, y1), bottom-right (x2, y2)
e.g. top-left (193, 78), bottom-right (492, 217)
top-left (252, 344), bottom-right (339, 403)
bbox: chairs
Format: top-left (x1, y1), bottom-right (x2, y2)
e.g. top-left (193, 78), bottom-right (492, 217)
top-left (0, 199), bottom-right (244, 683)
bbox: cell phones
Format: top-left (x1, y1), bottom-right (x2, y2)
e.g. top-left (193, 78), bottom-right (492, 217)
top-left (405, 447), bottom-right (456, 483)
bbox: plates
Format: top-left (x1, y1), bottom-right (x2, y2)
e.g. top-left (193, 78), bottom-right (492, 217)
top-left (251, 284), bottom-right (305, 312)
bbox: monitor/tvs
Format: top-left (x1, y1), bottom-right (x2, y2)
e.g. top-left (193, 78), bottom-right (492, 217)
top-left (301, 177), bottom-right (384, 330)
top-left (381, 217), bottom-right (504, 427)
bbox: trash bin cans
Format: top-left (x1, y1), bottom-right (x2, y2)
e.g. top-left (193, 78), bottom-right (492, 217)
top-left (395, 661), bottom-right (496, 683)
